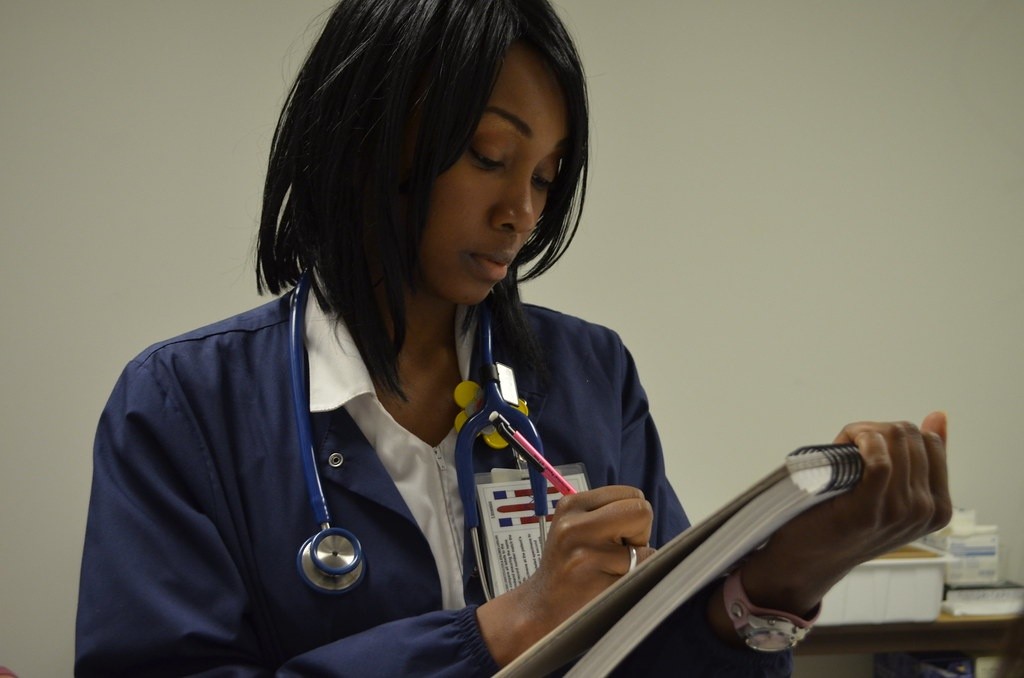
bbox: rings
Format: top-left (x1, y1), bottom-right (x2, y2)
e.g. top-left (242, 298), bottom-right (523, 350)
top-left (624, 542), bottom-right (640, 578)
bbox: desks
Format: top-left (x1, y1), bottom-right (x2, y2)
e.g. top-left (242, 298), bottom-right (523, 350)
top-left (789, 614), bottom-right (1024, 678)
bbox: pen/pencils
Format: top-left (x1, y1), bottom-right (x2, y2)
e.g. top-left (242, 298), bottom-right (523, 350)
top-left (488, 410), bottom-right (631, 546)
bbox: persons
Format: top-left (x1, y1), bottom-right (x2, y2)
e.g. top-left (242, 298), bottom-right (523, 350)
top-left (73, 0), bottom-right (953, 678)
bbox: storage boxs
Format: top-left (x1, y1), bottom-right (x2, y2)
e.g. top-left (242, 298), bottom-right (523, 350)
top-left (809, 540), bottom-right (960, 626)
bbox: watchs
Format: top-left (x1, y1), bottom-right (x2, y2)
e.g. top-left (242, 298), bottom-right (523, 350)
top-left (722, 573), bottom-right (822, 653)
